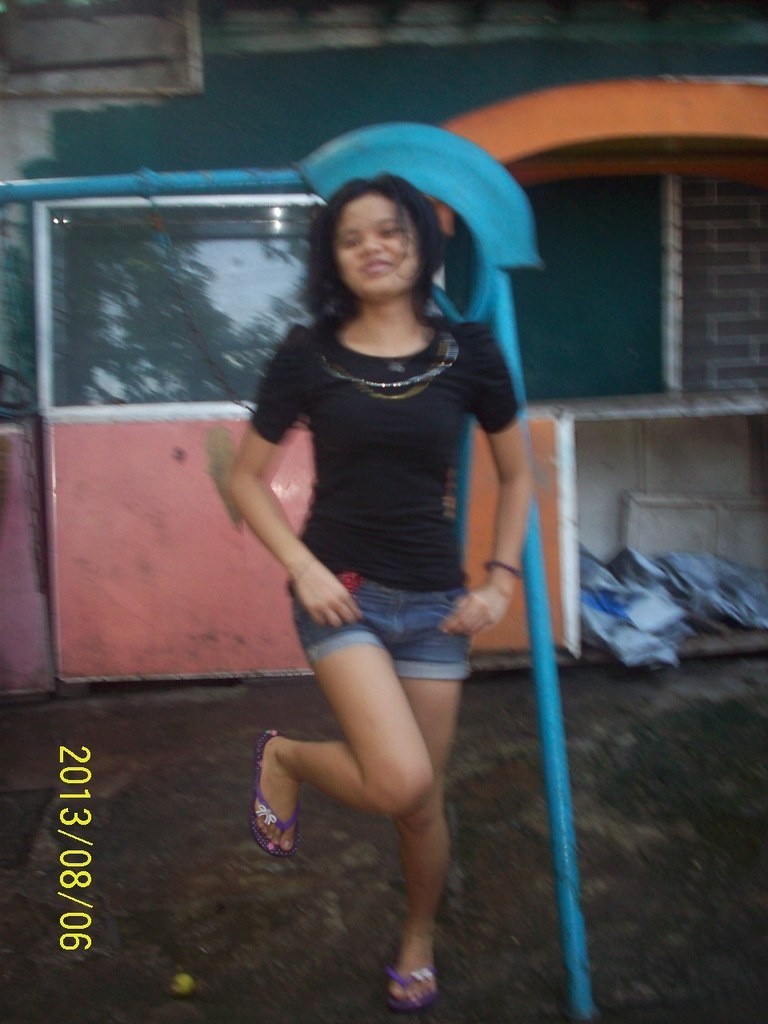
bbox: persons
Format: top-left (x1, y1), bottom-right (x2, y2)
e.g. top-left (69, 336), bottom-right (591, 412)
top-left (223, 170), bottom-right (534, 1008)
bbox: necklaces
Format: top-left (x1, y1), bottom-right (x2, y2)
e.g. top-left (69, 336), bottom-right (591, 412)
top-left (331, 335), bottom-right (448, 400)
top-left (313, 322), bottom-right (460, 388)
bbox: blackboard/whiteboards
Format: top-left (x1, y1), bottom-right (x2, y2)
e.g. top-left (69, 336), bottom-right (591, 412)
top-left (37, 26), bottom-right (684, 410)
top-left (34, 194), bottom-right (329, 420)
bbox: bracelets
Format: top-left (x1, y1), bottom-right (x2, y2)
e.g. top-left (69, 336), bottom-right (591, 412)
top-left (482, 560), bottom-right (522, 581)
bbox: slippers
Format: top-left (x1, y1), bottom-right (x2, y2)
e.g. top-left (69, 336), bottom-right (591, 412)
top-left (252, 729), bottom-right (304, 856)
top-left (386, 966), bottom-right (437, 1009)
